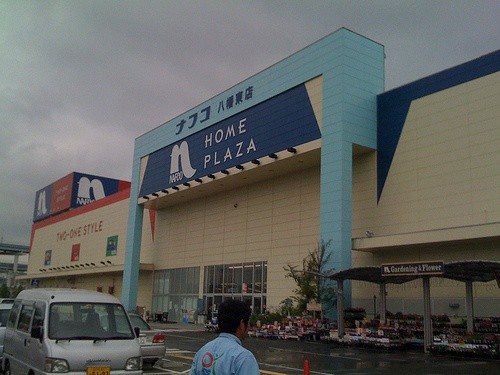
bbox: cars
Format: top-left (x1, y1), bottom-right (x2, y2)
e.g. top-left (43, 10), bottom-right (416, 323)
top-left (98, 310), bottom-right (167, 370)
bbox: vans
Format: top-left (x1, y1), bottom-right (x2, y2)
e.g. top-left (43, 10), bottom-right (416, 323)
top-left (0, 297), bottom-right (17, 361)
top-left (2, 286), bottom-right (143, 375)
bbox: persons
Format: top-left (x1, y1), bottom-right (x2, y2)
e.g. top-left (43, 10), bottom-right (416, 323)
top-left (188, 299), bottom-right (261, 375)
